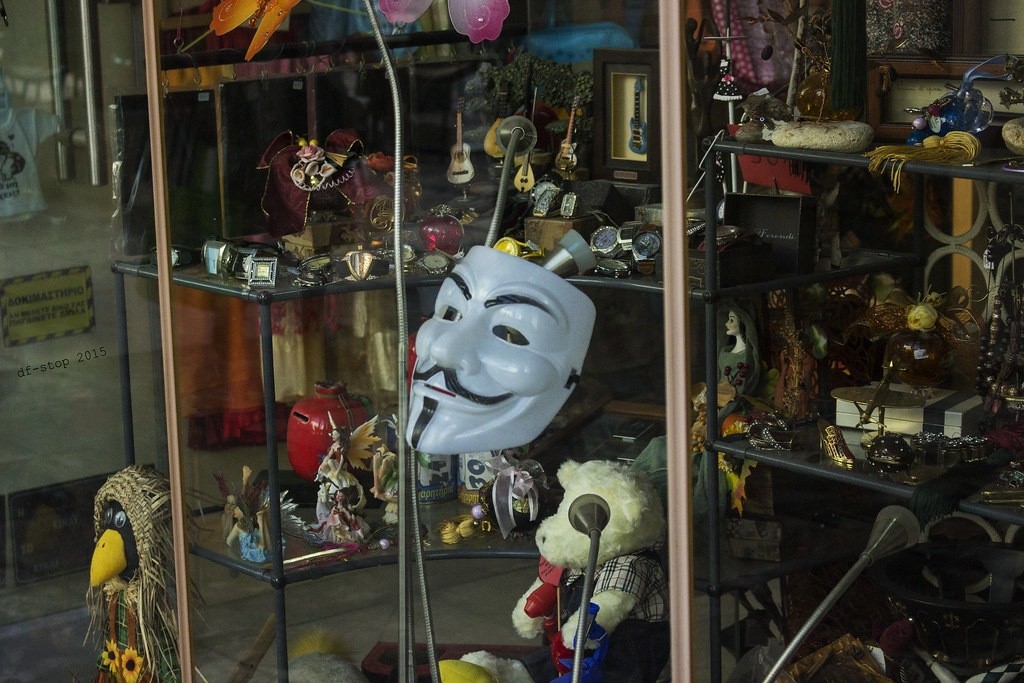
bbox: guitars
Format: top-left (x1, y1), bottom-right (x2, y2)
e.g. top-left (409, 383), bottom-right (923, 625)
top-left (556, 94), bottom-right (582, 172)
top-left (484, 79), bottom-right (510, 160)
top-left (513, 149), bottom-right (535, 193)
top-left (445, 97), bottom-right (477, 184)
top-left (627, 74), bottom-right (648, 155)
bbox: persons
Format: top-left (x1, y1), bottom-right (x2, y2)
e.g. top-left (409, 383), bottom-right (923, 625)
top-left (629, 302), bottom-right (761, 518)
top-left (225, 411), bottom-right (419, 556)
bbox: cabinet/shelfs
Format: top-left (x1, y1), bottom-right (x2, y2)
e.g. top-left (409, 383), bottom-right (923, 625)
top-left (79, 0), bottom-right (1024, 683)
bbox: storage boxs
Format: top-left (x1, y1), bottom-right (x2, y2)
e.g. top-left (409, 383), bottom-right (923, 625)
top-left (721, 190), bottom-right (817, 276)
top-left (833, 381), bottom-right (961, 438)
top-left (720, 516), bottom-right (800, 563)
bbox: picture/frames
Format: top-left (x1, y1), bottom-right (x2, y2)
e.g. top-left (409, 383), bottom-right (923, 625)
top-left (593, 47), bottom-right (660, 182)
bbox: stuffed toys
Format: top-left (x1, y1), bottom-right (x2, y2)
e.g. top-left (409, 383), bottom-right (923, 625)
top-left (276, 625), bottom-right (370, 683)
top-left (438, 460), bottom-right (669, 683)
